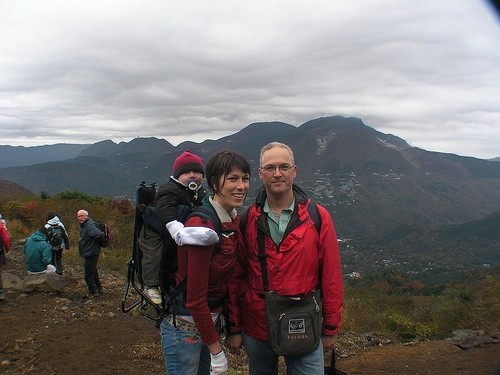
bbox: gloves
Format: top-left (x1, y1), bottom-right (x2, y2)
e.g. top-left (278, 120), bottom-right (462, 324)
top-left (210, 349), bottom-right (229, 375)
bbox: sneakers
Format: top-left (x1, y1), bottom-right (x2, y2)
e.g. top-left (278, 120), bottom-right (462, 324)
top-left (88, 291), bottom-right (99, 299)
top-left (0, 287), bottom-right (5, 294)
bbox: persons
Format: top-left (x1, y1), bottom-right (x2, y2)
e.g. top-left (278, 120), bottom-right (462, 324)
top-left (76, 209), bottom-right (105, 298)
top-left (0, 214), bottom-right (10, 299)
top-left (160, 150), bottom-right (250, 375)
top-left (23, 226), bottom-right (52, 276)
top-left (224, 142), bottom-right (344, 375)
top-left (45, 211), bottom-right (70, 275)
top-left (138, 150), bottom-right (219, 304)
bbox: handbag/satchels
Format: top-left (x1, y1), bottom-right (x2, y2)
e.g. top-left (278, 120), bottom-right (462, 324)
top-left (324, 348), bottom-right (347, 375)
top-left (264, 289), bottom-right (322, 355)
top-left (143, 285), bottom-right (162, 304)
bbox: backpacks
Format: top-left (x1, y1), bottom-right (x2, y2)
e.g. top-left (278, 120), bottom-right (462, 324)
top-left (94, 219), bottom-right (109, 247)
top-left (46, 223), bottom-right (62, 245)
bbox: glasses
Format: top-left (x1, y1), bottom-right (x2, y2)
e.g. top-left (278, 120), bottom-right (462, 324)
top-left (261, 164), bottom-right (296, 171)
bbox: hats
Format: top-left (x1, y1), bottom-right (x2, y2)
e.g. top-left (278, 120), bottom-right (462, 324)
top-left (174, 150), bottom-right (205, 180)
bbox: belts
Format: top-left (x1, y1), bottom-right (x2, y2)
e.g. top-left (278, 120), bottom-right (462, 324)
top-left (165, 314), bottom-right (218, 332)
